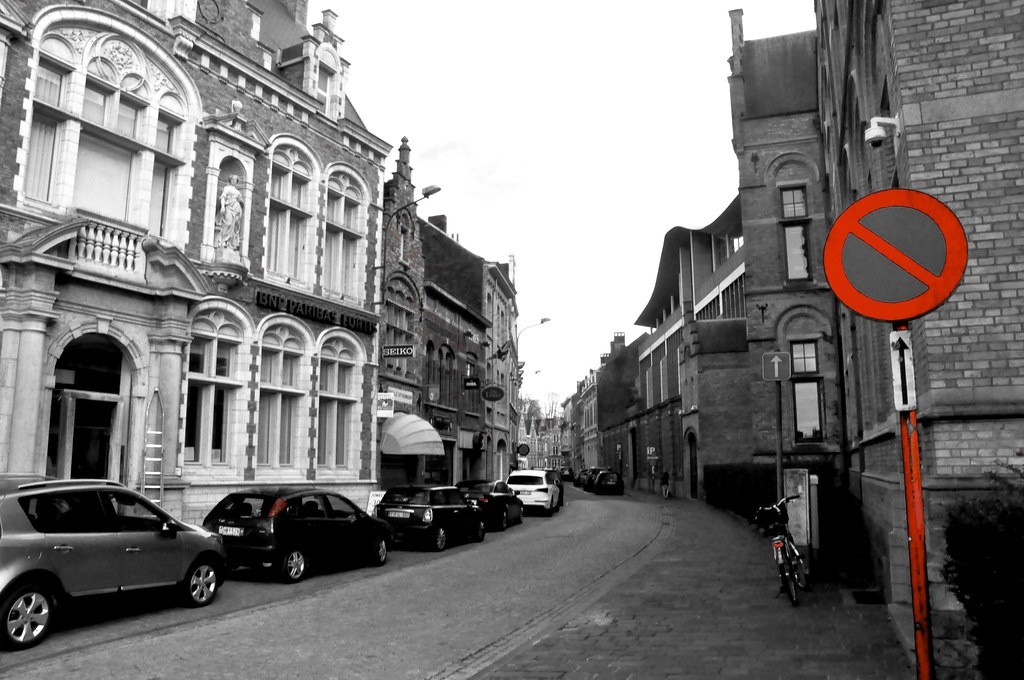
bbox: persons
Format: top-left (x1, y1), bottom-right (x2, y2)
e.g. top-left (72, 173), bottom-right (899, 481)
top-left (660, 472), bottom-right (669, 498)
top-left (509, 463), bottom-right (516, 475)
top-left (442, 465), bottom-right (449, 485)
top-left (608, 466), bottom-right (612, 471)
top-left (218, 175), bottom-right (244, 249)
top-left (569, 467), bottom-right (575, 482)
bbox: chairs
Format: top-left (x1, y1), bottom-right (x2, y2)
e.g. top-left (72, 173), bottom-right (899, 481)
top-left (302, 500), bottom-right (320, 517)
top-left (231, 501), bottom-right (253, 517)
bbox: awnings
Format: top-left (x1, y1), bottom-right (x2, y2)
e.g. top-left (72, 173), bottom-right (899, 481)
top-left (380, 413), bottom-right (445, 455)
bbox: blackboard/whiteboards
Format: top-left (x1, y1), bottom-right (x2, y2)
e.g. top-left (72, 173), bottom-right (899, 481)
top-left (366, 491), bottom-right (387, 516)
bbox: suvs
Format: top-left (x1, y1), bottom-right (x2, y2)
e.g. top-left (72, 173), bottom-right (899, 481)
top-left (0, 474), bottom-right (230, 654)
top-left (583, 466), bottom-right (612, 492)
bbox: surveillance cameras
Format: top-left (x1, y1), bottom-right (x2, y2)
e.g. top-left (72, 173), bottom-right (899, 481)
top-left (865, 126), bottom-right (886, 148)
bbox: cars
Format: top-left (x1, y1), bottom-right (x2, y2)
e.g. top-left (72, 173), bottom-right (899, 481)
top-left (593, 471), bottom-right (625, 497)
top-left (372, 484), bottom-right (488, 553)
top-left (573, 468), bottom-right (589, 487)
top-left (201, 489), bottom-right (394, 584)
top-left (532, 467), bottom-right (565, 507)
top-left (455, 479), bottom-right (525, 533)
top-left (505, 470), bottom-right (562, 518)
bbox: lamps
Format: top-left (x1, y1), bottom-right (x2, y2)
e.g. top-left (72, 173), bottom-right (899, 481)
top-left (516, 318), bottom-right (550, 340)
top-left (370, 184), bottom-right (441, 307)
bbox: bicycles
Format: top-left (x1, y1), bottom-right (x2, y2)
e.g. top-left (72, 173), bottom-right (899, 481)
top-left (756, 495), bottom-right (812, 606)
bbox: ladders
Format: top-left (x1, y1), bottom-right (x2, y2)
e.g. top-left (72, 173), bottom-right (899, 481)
top-left (140, 389), bottom-right (164, 508)
